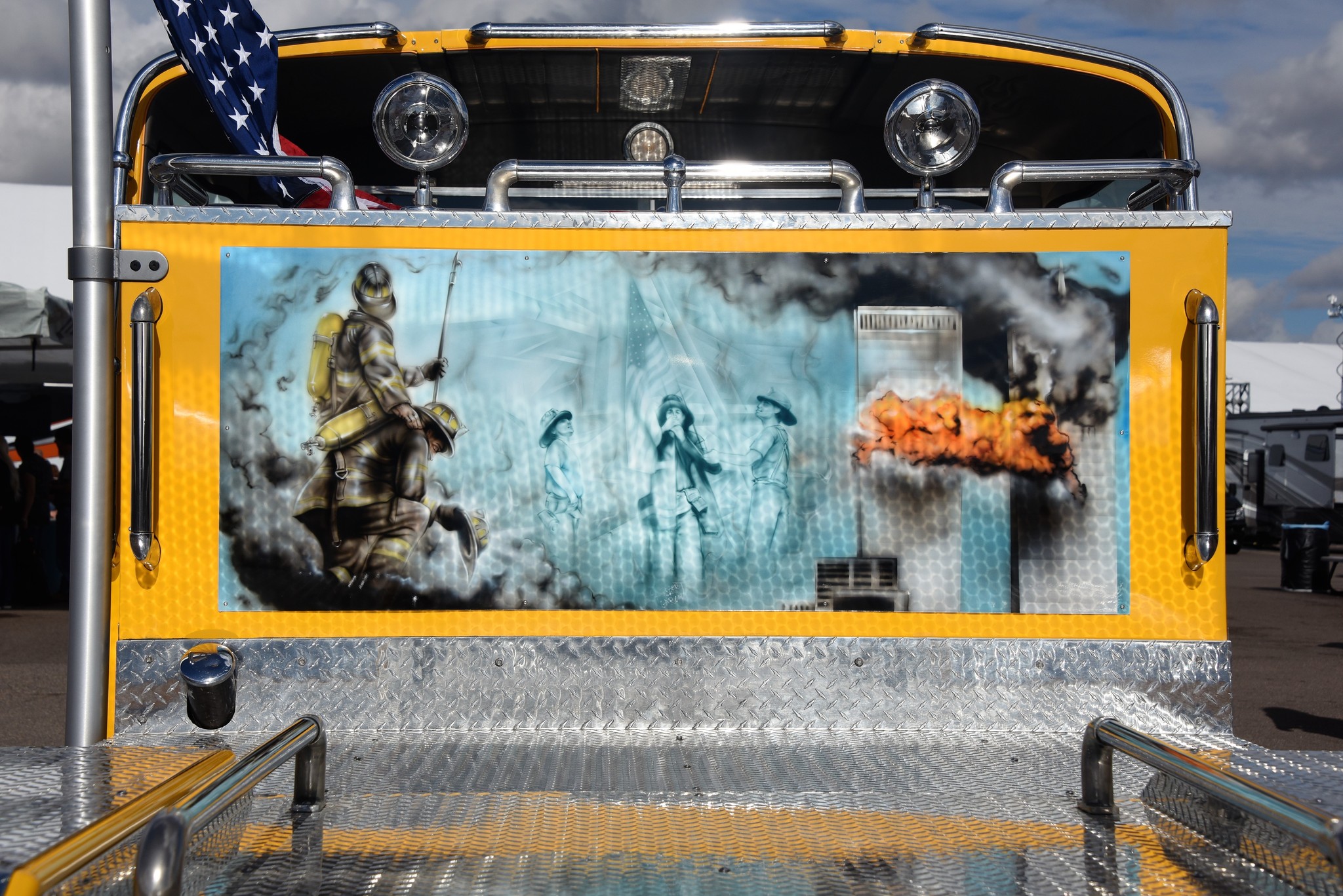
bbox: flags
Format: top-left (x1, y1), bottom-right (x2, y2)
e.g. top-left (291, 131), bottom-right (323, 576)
top-left (150, 0), bottom-right (409, 214)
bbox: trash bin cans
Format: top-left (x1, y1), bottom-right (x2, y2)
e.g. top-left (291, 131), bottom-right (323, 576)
top-left (1278, 510), bottom-right (1333, 596)
